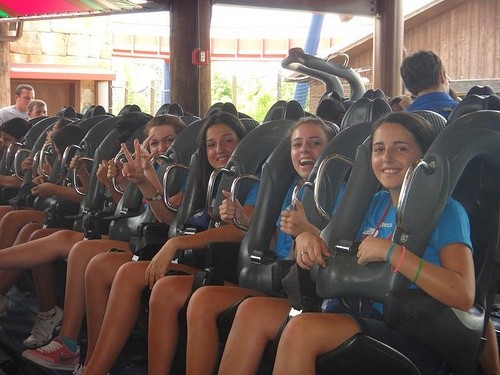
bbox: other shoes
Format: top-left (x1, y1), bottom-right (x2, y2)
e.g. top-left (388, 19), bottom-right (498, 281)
top-left (0, 294), bottom-right (18, 318)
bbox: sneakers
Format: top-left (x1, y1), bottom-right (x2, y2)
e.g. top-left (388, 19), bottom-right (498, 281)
top-left (22, 337), bottom-right (81, 371)
top-left (23, 304), bottom-right (64, 349)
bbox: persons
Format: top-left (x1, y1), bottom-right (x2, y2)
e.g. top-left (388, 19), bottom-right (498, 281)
top-left (0, 112), bottom-right (188, 375)
top-left (216, 114), bottom-right (476, 375)
top-left (388, 49), bottom-right (500, 375)
top-left (146, 116), bottom-right (349, 375)
top-left (0, 84), bottom-right (49, 128)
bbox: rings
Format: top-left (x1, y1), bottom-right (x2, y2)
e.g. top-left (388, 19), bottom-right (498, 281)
top-left (283, 216), bottom-right (287, 223)
top-left (300, 251), bottom-right (308, 256)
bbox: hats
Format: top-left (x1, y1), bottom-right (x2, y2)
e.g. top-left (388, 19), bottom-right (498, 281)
top-left (0, 118), bottom-right (32, 140)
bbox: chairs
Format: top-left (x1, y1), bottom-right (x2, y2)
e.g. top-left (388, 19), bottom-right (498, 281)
top-left (0, 48), bottom-right (500, 375)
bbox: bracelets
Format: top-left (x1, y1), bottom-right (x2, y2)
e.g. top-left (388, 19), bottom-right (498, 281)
top-left (385, 242), bottom-right (398, 265)
top-left (390, 246), bottom-right (406, 273)
top-left (409, 258), bottom-right (424, 286)
top-left (146, 193), bottom-right (162, 201)
